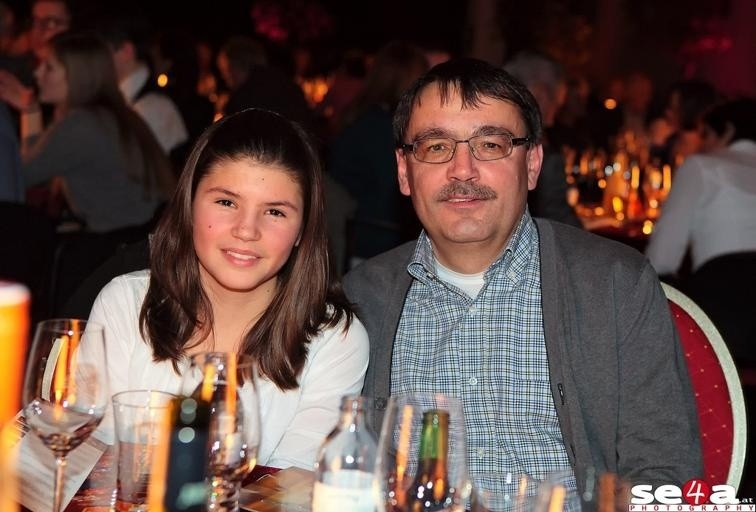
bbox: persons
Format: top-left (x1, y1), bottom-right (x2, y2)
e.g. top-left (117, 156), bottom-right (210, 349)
top-left (336, 55), bottom-right (705, 510)
top-left (60, 105), bottom-right (372, 474)
top-left (3, 1), bottom-right (753, 425)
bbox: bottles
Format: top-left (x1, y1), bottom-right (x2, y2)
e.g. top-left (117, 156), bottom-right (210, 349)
top-left (392, 408), bottom-right (460, 512)
top-left (310, 394), bottom-right (387, 512)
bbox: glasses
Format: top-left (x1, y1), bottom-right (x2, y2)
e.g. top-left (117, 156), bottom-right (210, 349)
top-left (400, 134), bottom-right (532, 164)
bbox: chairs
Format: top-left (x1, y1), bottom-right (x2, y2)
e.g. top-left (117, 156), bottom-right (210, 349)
top-left (657, 279), bottom-right (748, 504)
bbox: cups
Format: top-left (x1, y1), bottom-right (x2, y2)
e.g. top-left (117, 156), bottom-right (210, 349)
top-left (373, 394), bottom-right (473, 511)
top-left (110, 390), bottom-right (184, 509)
top-left (569, 144), bottom-right (673, 227)
top-left (476, 472), bottom-right (682, 511)
top-left (180, 352), bottom-right (261, 511)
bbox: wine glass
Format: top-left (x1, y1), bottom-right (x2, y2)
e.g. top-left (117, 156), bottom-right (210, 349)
top-left (22, 319), bottom-right (108, 510)
